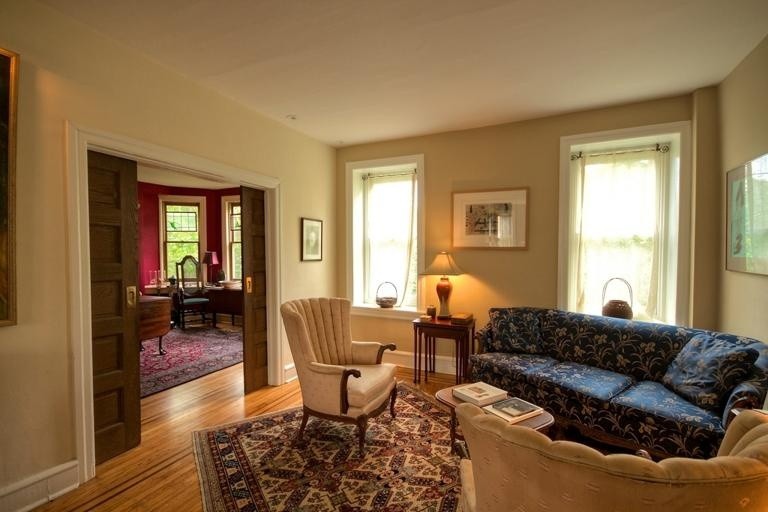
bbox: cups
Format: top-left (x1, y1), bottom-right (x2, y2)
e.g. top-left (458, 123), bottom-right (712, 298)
top-left (148, 269), bottom-right (166, 287)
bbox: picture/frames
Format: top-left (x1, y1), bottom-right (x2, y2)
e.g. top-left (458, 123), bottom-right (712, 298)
top-left (725, 152), bottom-right (768, 277)
top-left (451, 187), bottom-right (530, 251)
top-left (0, 48), bottom-right (20, 327)
top-left (300, 217), bottom-right (323, 261)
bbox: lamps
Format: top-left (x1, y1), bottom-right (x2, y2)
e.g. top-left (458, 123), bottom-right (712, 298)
top-left (202, 251), bottom-right (219, 287)
top-left (419, 251), bottom-right (464, 320)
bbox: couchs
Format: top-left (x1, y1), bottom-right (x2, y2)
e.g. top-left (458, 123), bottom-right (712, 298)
top-left (453, 403), bottom-right (768, 512)
top-left (468, 307), bottom-right (768, 463)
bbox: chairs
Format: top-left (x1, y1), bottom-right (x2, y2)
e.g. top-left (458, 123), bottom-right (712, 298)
top-left (280, 298), bottom-right (398, 459)
top-left (175, 255), bottom-right (216, 334)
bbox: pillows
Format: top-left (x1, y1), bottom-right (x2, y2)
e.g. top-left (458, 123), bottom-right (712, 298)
top-left (488, 308), bottom-right (546, 356)
top-left (661, 332), bottom-right (760, 418)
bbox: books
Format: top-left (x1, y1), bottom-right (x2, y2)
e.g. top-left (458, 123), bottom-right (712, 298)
top-left (482, 397), bottom-right (544, 425)
top-left (450, 311), bottom-right (474, 325)
top-left (492, 398), bottom-right (538, 417)
top-left (451, 381), bottom-right (508, 407)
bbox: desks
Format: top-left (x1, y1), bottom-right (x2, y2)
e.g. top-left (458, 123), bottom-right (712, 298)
top-left (145, 282), bottom-right (243, 328)
top-left (138, 295), bottom-right (171, 355)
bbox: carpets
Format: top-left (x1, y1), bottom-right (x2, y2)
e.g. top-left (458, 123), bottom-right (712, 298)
top-left (140, 325), bottom-right (244, 399)
top-left (190, 381), bottom-right (464, 512)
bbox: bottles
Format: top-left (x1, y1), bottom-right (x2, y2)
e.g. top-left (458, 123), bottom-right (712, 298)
top-left (426, 303), bottom-right (436, 317)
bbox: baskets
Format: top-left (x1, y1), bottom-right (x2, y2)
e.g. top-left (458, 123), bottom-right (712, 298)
top-left (374, 280), bottom-right (399, 308)
top-left (600, 275), bottom-right (634, 320)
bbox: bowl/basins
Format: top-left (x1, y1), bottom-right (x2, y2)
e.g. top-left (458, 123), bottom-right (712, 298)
top-left (218, 280), bottom-right (240, 289)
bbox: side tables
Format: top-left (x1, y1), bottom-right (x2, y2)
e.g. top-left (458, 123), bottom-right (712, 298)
top-left (725, 407), bottom-right (752, 431)
top-left (412, 315), bottom-right (477, 384)
